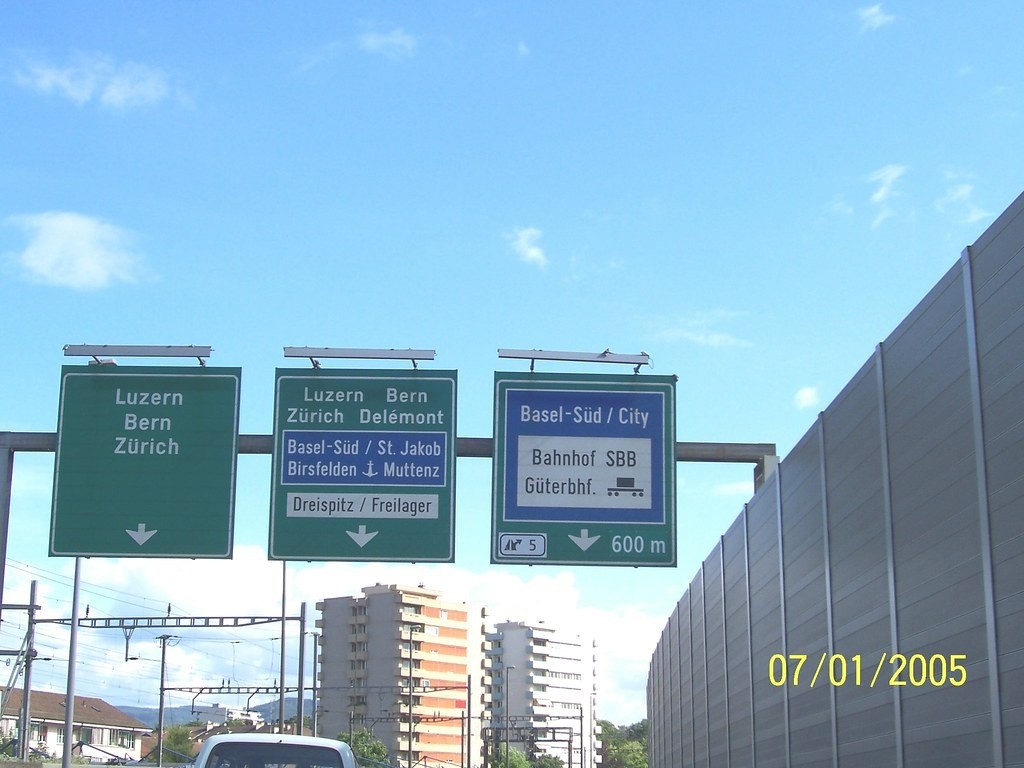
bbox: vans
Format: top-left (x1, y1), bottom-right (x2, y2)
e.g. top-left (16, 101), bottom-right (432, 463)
top-left (193, 733), bottom-right (358, 768)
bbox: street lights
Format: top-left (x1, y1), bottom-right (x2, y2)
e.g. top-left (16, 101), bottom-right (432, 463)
top-left (506, 665), bottom-right (516, 768)
top-left (408, 625), bottom-right (421, 768)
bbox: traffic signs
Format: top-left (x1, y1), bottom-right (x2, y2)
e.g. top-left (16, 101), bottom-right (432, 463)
top-left (490, 369), bottom-right (679, 567)
top-left (268, 367), bottom-right (459, 563)
top-left (48, 364), bottom-right (242, 560)
